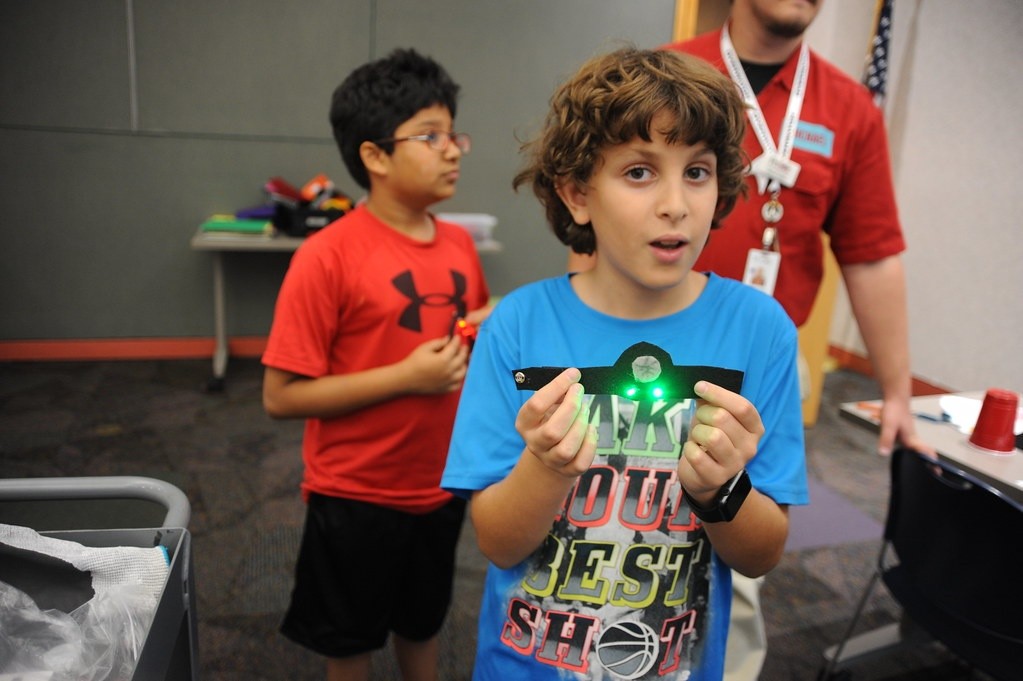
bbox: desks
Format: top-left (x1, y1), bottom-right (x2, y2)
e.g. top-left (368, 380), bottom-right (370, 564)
top-left (824, 390), bottom-right (1023, 681)
top-left (196, 213), bottom-right (503, 394)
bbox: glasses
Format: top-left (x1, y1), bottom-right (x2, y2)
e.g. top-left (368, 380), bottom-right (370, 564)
top-left (376, 130), bottom-right (472, 155)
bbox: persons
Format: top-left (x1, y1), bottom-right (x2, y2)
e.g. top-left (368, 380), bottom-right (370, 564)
top-left (441, 49), bottom-right (813, 681)
top-left (656, 0), bottom-right (944, 474)
top-left (263, 47), bottom-right (495, 681)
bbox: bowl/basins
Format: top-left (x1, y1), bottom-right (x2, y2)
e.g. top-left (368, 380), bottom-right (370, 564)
top-left (438, 210), bottom-right (498, 243)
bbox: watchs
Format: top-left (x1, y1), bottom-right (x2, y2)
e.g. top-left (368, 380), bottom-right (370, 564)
top-left (680, 470), bottom-right (752, 523)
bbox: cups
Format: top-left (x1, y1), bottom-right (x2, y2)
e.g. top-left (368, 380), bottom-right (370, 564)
top-left (968, 388), bottom-right (1019, 457)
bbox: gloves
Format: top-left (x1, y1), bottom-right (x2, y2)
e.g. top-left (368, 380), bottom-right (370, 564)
top-left (0, 523), bottom-right (170, 615)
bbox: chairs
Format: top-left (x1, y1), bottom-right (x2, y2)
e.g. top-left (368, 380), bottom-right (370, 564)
top-left (818, 447), bottom-right (1023, 681)
